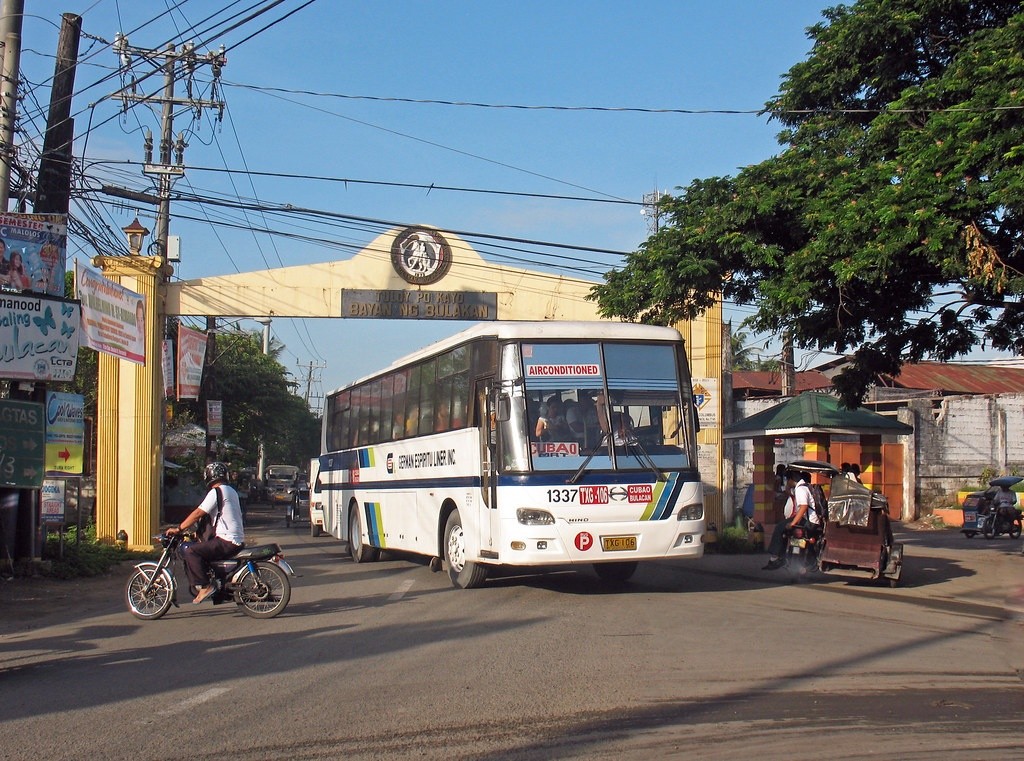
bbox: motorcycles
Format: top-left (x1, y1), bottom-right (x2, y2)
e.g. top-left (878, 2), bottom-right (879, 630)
top-left (125, 523), bottom-right (305, 621)
top-left (783, 524), bottom-right (825, 584)
top-left (977, 500), bottom-right (1024, 539)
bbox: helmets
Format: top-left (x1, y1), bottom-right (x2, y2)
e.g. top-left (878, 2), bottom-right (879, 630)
top-left (203, 462), bottom-right (229, 487)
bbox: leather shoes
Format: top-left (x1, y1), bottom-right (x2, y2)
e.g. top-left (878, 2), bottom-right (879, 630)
top-left (761, 558), bottom-right (785, 571)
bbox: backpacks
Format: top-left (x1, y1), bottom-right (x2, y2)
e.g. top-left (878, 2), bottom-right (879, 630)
top-left (798, 482), bottom-right (829, 525)
top-left (784, 493), bottom-right (796, 519)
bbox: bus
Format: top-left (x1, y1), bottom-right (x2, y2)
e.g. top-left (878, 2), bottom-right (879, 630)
top-left (319, 321), bottom-right (707, 591)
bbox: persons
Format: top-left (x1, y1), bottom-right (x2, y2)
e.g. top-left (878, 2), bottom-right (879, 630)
top-left (164, 463), bottom-right (243, 604)
top-left (419, 242), bottom-right (426, 256)
top-left (990, 485), bottom-right (1016, 517)
top-left (0, 238), bottom-right (29, 290)
top-left (535, 396), bottom-right (594, 442)
top-left (133, 299), bottom-right (145, 354)
top-left (760, 462), bottom-right (864, 571)
top-left (601, 414), bottom-right (638, 446)
top-left (436, 400), bottom-right (449, 430)
top-left (391, 411), bottom-right (418, 439)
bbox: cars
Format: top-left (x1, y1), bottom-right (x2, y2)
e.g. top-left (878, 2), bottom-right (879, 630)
top-left (266, 465), bottom-right (310, 527)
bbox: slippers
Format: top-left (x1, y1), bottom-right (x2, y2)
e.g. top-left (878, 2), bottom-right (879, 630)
top-left (193, 587), bottom-right (216, 605)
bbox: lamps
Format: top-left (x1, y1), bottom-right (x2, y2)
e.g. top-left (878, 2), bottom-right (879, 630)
top-left (121, 219), bottom-right (150, 257)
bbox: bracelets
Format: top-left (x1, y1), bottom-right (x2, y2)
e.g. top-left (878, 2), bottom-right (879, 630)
top-left (178, 525), bottom-right (183, 532)
